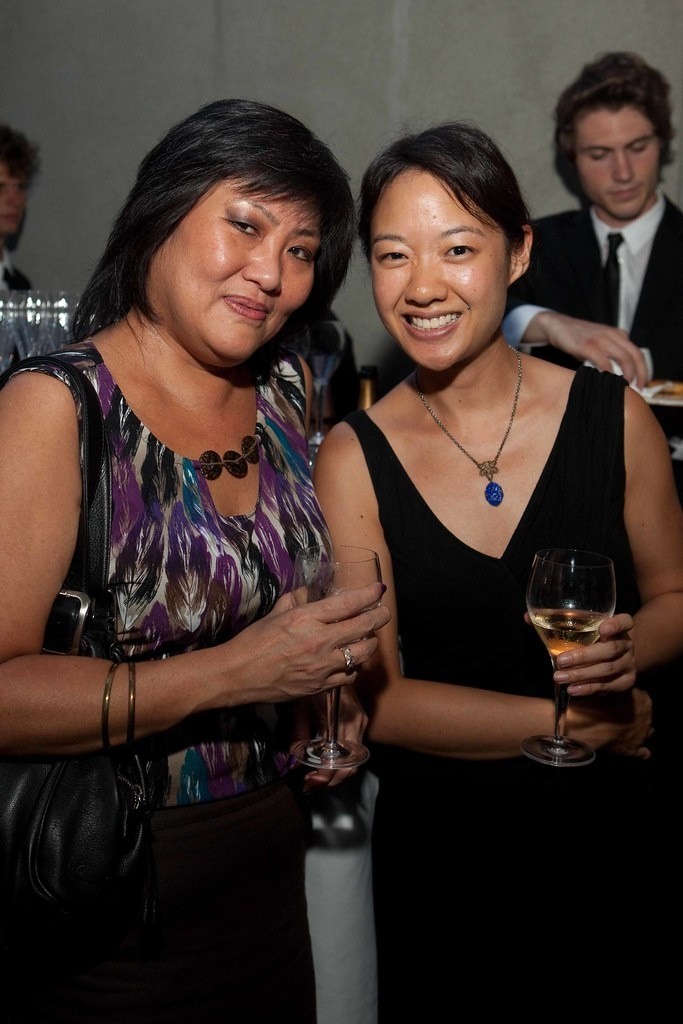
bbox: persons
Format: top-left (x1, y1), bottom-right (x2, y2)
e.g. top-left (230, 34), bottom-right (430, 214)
top-left (1, 120), bottom-right (39, 296)
top-left (306, 117), bottom-right (681, 1023)
top-left (499, 50), bottom-right (682, 498)
top-left (300, 301), bottom-right (362, 445)
top-left (2, 96), bottom-right (393, 1022)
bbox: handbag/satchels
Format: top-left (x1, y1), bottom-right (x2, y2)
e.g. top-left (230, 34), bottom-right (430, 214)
top-left (1, 660), bottom-right (163, 910)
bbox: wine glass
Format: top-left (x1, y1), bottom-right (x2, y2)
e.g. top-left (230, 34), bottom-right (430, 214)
top-left (517, 548), bottom-right (619, 774)
top-left (2, 285), bottom-right (86, 371)
top-left (287, 545), bottom-right (383, 773)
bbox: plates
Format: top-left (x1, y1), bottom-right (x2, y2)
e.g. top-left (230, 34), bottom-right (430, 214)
top-left (638, 383), bottom-right (681, 408)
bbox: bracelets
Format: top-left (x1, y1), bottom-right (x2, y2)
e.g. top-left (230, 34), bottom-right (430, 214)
top-left (101, 661), bottom-right (121, 747)
top-left (125, 663), bottom-right (137, 748)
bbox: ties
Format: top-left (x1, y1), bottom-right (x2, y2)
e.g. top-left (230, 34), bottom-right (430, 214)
top-left (603, 232), bottom-right (625, 328)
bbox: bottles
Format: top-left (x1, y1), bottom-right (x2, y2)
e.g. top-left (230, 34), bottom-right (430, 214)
top-left (359, 364), bottom-right (380, 415)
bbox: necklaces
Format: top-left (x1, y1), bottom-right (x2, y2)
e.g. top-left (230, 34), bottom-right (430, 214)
top-left (410, 343), bottom-right (525, 507)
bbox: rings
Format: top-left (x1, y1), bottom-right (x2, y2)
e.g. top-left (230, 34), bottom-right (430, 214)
top-left (339, 645), bottom-right (355, 675)
